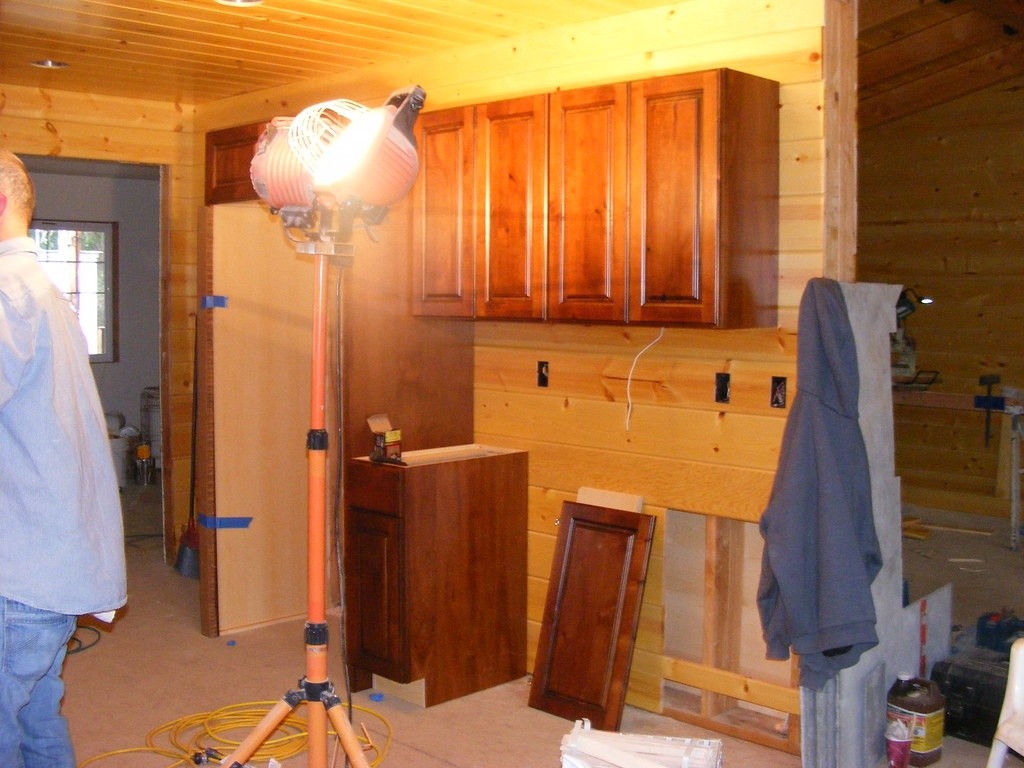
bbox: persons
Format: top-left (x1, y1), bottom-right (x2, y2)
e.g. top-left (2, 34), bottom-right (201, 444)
top-left (0, 151), bottom-right (129, 768)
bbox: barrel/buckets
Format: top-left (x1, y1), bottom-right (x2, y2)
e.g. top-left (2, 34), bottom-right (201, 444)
top-left (135, 459), bottom-right (155, 486)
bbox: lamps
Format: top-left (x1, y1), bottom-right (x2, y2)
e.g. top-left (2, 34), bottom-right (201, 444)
top-left (218, 88), bottom-right (431, 768)
top-left (902, 286), bottom-right (934, 307)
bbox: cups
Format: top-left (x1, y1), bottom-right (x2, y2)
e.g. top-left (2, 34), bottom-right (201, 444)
top-left (884, 731), bottom-right (912, 768)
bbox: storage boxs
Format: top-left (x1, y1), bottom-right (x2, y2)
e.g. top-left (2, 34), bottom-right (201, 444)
top-left (934, 656), bottom-right (1009, 744)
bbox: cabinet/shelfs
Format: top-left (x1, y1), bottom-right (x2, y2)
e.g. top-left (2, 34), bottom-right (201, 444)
top-left (408, 69), bottom-right (781, 332)
top-left (350, 446), bottom-right (529, 710)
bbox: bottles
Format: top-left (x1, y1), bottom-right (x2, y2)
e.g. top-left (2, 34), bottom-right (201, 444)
top-left (136, 445), bottom-right (151, 459)
top-left (885, 670), bottom-right (946, 767)
